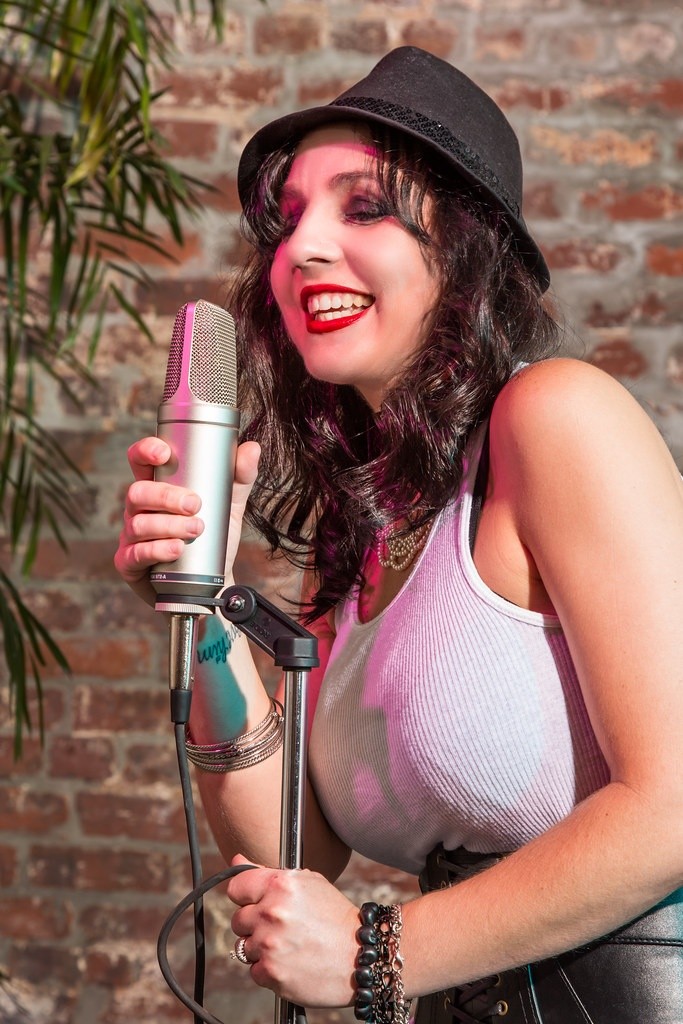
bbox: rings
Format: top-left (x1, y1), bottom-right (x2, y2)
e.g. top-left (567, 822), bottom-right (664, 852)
top-left (229, 934), bottom-right (254, 964)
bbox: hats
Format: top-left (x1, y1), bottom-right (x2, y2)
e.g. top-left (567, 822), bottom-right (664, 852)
top-left (238, 46), bottom-right (551, 300)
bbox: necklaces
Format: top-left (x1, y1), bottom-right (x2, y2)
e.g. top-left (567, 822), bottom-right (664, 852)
top-left (376, 515), bottom-right (432, 571)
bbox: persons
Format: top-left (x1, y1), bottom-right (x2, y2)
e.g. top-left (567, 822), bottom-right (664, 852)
top-left (114, 46), bottom-right (683, 1024)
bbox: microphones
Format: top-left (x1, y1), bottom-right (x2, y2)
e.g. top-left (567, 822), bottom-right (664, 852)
top-left (149, 301), bottom-right (242, 725)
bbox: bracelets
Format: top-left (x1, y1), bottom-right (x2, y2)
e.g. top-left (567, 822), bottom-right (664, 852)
top-left (353, 902), bottom-right (412, 1024)
top-left (187, 696), bottom-right (291, 772)
